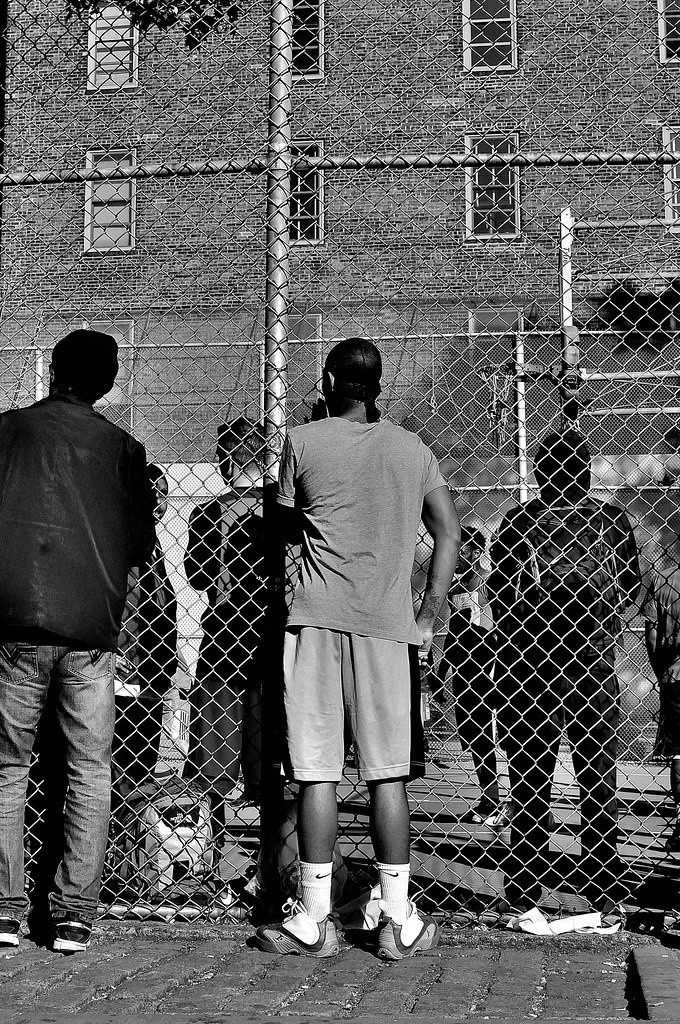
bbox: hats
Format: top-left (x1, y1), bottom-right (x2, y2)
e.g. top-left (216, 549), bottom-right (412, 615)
top-left (533, 431), bottom-right (590, 490)
top-left (323, 338), bottom-right (382, 384)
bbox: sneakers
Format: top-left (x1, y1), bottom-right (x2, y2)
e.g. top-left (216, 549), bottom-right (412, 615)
top-left (0, 916), bottom-right (21, 947)
top-left (377, 898), bottom-right (440, 959)
top-left (53, 910), bottom-right (93, 952)
top-left (255, 896), bottom-right (339, 958)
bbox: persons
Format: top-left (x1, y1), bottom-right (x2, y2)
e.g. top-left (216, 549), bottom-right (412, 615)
top-left (117, 466), bottom-right (179, 783)
top-left (185, 418), bottom-right (265, 846)
top-left (485, 429), bottom-right (640, 854)
top-left (0, 330), bottom-right (158, 951)
top-left (436, 526), bottom-right (499, 823)
top-left (639, 566), bottom-right (680, 852)
top-left (255, 337), bottom-right (461, 959)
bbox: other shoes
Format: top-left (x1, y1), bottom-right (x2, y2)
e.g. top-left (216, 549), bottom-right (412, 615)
top-left (460, 800), bottom-right (503, 825)
top-left (478, 899), bottom-right (528, 917)
top-left (485, 801), bottom-right (516, 827)
top-left (549, 812), bottom-right (558, 827)
top-left (665, 819), bottom-right (680, 852)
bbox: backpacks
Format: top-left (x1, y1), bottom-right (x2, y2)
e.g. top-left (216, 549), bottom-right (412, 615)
top-left (515, 498), bottom-right (617, 646)
top-left (109, 766), bottom-right (216, 906)
